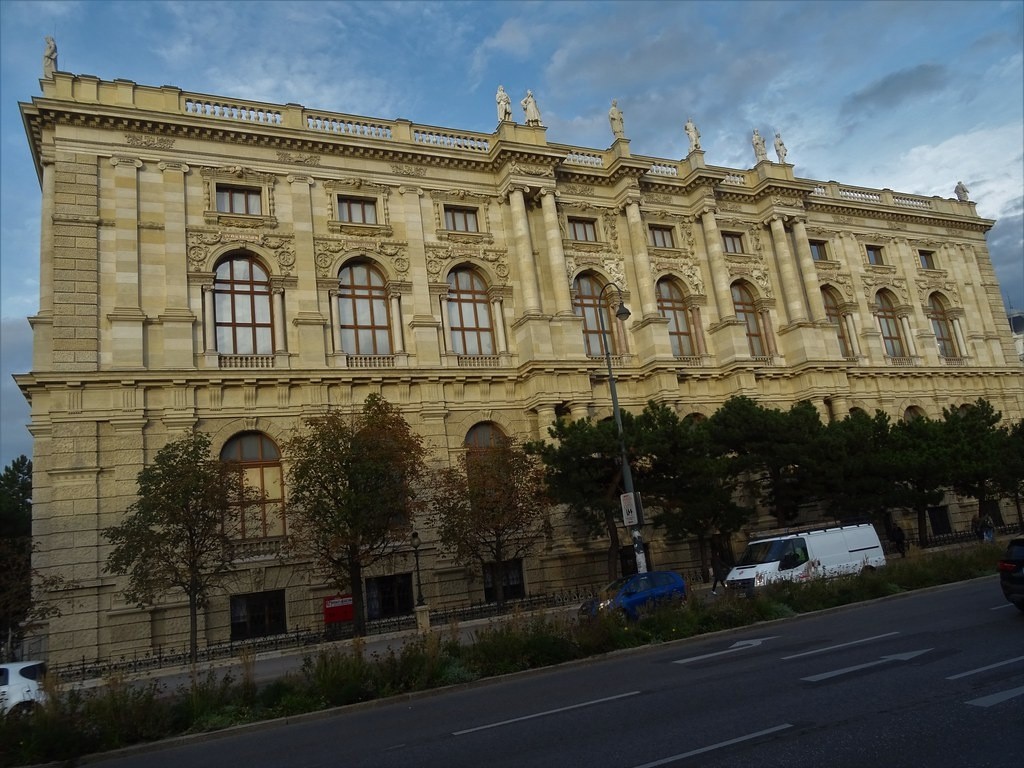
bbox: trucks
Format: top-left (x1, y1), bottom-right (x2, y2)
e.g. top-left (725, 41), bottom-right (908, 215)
top-left (722, 522), bottom-right (885, 604)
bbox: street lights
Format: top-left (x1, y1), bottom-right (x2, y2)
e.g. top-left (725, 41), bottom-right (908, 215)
top-left (598, 283), bottom-right (648, 573)
top-left (410, 532), bottom-right (427, 606)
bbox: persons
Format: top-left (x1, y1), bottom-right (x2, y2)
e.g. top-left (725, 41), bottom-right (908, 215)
top-left (609, 99), bottom-right (624, 138)
top-left (521, 89), bottom-right (543, 127)
top-left (892, 523), bottom-right (906, 558)
top-left (774, 132), bottom-right (787, 165)
top-left (970, 511), bottom-right (993, 544)
top-left (751, 129), bottom-right (767, 162)
top-left (709, 550), bottom-right (732, 593)
top-left (684, 118), bottom-right (701, 153)
top-left (43, 35), bottom-right (58, 79)
top-left (954, 181), bottom-right (969, 202)
top-left (496, 85), bottom-right (512, 123)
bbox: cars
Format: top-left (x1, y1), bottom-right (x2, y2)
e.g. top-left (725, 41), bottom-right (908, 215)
top-left (0, 661), bottom-right (52, 730)
top-left (577, 572), bottom-right (687, 626)
top-left (998, 534), bottom-right (1024, 616)
